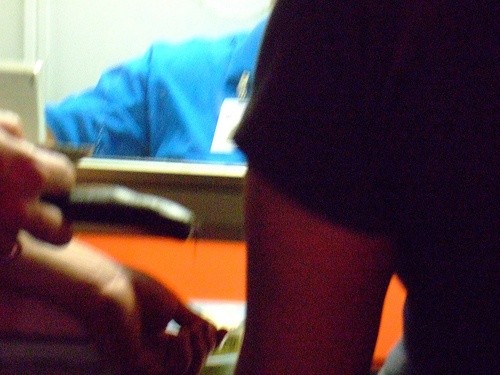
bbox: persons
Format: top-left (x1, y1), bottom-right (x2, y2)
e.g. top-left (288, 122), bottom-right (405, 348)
top-left (230, 0), bottom-right (499, 375)
top-left (0, 109), bottom-right (226, 375)
top-left (40, 13), bottom-right (270, 162)
